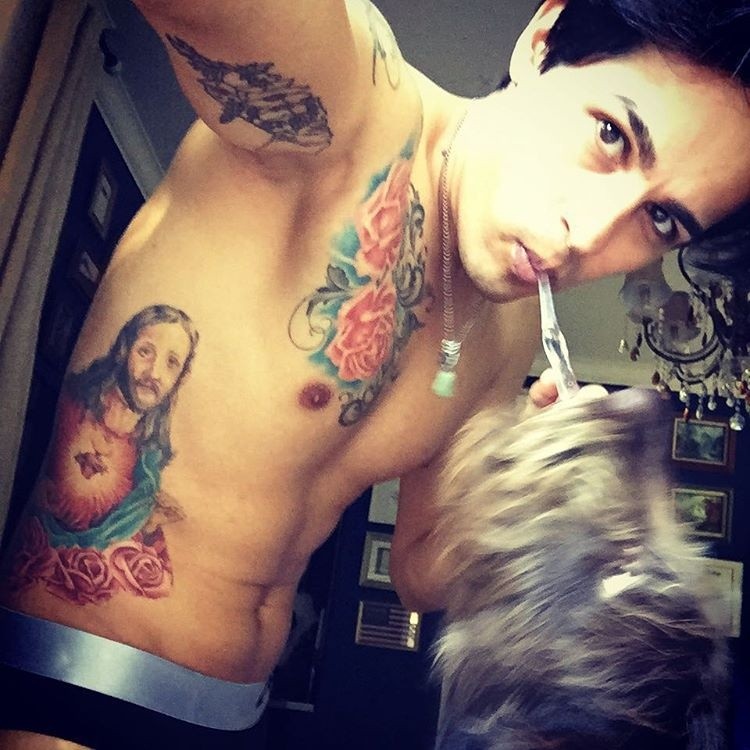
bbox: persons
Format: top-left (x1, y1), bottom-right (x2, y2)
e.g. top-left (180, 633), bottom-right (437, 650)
top-left (2, 2), bottom-right (750, 748)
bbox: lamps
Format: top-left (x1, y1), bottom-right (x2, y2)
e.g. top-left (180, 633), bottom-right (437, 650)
top-left (617, 230), bottom-right (750, 432)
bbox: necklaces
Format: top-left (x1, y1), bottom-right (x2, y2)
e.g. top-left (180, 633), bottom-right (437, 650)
top-left (432, 94), bottom-right (497, 398)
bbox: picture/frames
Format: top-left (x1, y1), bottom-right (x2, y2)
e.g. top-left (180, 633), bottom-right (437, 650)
top-left (84, 156), bottom-right (119, 241)
top-left (671, 481), bottom-right (733, 545)
top-left (689, 552), bottom-right (742, 639)
top-left (359, 532), bottom-right (396, 592)
top-left (666, 411), bottom-right (735, 474)
top-left (355, 600), bottom-right (421, 652)
